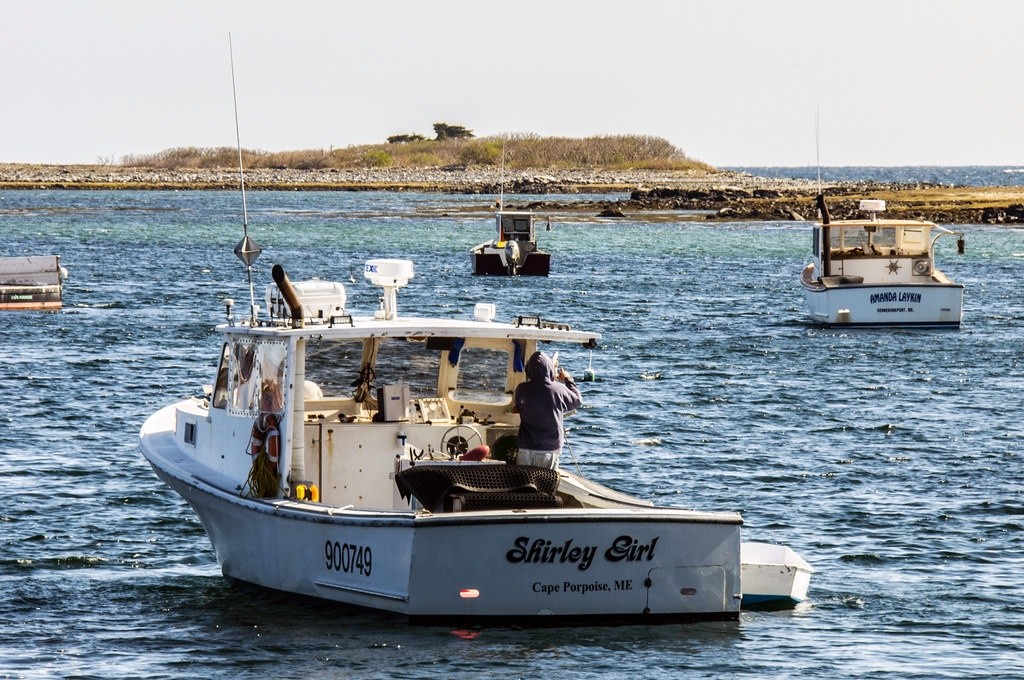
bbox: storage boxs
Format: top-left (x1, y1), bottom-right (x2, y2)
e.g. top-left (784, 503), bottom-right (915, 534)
top-left (266, 281), bottom-right (345, 318)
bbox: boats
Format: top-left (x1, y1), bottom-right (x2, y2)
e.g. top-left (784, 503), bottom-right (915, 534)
top-left (0, 255), bottom-right (68, 310)
top-left (468, 140), bottom-right (552, 278)
top-left (134, 33), bottom-right (813, 630)
top-left (795, 191), bottom-right (968, 329)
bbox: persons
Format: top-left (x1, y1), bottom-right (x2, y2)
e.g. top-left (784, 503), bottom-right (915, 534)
top-left (512, 351), bottom-right (583, 473)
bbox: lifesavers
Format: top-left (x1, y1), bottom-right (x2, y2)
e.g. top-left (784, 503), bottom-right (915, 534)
top-left (249, 414), bottom-right (279, 471)
